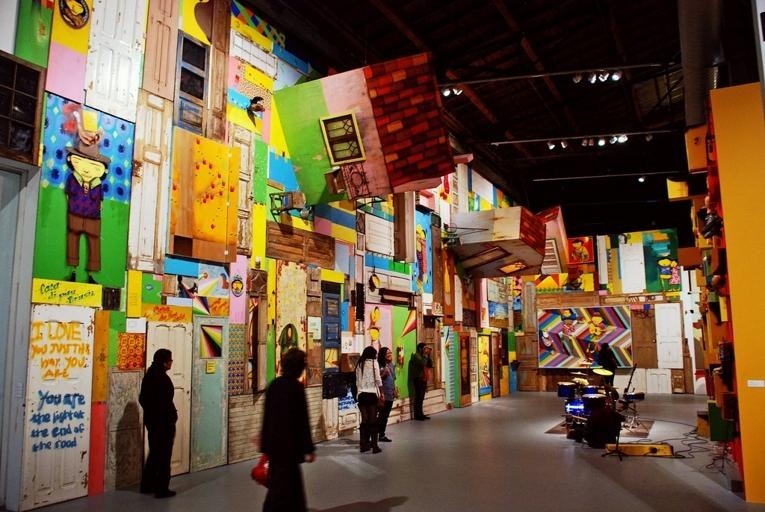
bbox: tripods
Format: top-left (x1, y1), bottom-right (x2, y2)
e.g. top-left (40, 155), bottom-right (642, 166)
top-left (601, 427), bottom-right (629, 462)
top-left (708, 422), bottom-right (739, 475)
top-left (622, 400), bottom-right (647, 429)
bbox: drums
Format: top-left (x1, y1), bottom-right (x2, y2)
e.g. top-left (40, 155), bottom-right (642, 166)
top-left (558, 382), bottom-right (576, 397)
top-left (582, 385), bottom-right (600, 394)
top-left (564, 398), bottom-right (583, 426)
top-left (582, 394), bottom-right (608, 414)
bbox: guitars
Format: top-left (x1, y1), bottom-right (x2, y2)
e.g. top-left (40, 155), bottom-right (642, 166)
top-left (561, 414), bottom-right (591, 424)
top-left (617, 363), bottom-right (641, 412)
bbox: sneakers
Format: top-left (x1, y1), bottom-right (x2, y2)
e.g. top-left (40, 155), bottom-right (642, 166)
top-left (140, 485), bottom-right (176, 498)
top-left (360, 437), bottom-right (392, 453)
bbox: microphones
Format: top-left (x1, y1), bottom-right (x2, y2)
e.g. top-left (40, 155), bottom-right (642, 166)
top-left (632, 362), bottom-right (638, 373)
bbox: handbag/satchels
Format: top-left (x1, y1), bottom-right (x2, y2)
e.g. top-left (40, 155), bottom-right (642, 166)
top-left (250, 453), bottom-right (270, 489)
top-left (393, 386), bottom-right (400, 398)
top-left (378, 393), bottom-right (385, 407)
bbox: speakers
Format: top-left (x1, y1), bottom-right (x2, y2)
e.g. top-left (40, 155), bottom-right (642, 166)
top-left (720, 392), bottom-right (738, 422)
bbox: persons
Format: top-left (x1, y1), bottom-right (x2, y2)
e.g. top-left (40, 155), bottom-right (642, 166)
top-left (261, 347), bottom-right (316, 512)
top-left (177, 276), bottom-right (196, 298)
top-left (139, 349), bottom-right (176, 498)
top-left (413, 343), bottom-right (432, 420)
top-left (355, 346), bottom-right (399, 454)
top-left (599, 343), bottom-right (618, 386)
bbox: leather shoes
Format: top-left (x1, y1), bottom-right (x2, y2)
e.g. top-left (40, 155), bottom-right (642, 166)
top-left (415, 415), bottom-right (431, 421)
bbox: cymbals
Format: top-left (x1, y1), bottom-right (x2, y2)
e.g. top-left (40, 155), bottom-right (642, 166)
top-left (604, 384), bottom-right (618, 401)
top-left (592, 368), bottom-right (613, 376)
top-left (570, 371), bottom-right (588, 376)
